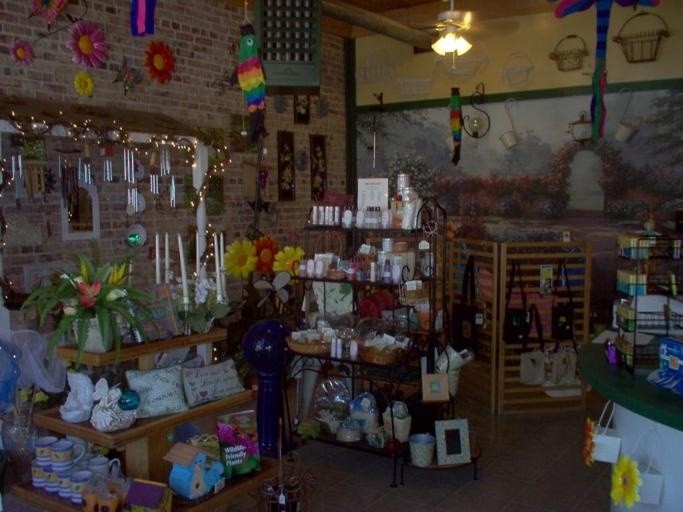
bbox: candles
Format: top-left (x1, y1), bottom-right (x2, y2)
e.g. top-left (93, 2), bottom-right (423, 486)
top-left (175, 232), bottom-right (190, 302)
top-left (212, 230), bottom-right (223, 302)
top-left (163, 230), bottom-right (172, 286)
top-left (153, 231), bottom-right (163, 287)
top-left (218, 232), bottom-right (228, 297)
top-left (194, 229), bottom-right (204, 283)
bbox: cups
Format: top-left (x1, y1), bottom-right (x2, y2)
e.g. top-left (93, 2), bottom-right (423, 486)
top-left (30, 434), bottom-right (121, 505)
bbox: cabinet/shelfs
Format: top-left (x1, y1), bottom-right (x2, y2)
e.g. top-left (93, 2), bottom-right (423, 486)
top-left (284, 193), bottom-right (487, 493)
top-left (447, 216), bottom-right (682, 425)
top-left (11, 325), bottom-right (284, 511)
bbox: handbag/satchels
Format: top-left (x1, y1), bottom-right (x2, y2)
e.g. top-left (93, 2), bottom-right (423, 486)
top-left (551, 347), bottom-right (578, 385)
top-left (503, 307), bottom-right (526, 343)
top-left (520, 350), bottom-right (544, 385)
top-left (450, 304), bottom-right (479, 350)
top-left (551, 304), bottom-right (575, 342)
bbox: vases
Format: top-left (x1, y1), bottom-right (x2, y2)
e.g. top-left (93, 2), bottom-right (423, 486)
top-left (69, 313), bottom-right (118, 354)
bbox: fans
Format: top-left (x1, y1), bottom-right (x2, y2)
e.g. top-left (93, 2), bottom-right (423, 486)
top-left (394, 1), bottom-right (519, 53)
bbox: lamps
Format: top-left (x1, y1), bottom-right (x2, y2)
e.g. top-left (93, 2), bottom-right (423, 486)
top-left (568, 110), bottom-right (590, 145)
top-left (430, 29), bottom-right (473, 55)
top-left (462, 82), bottom-right (490, 138)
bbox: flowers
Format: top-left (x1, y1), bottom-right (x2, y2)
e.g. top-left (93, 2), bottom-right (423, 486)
top-left (225, 233), bottom-right (309, 292)
top-left (579, 417), bottom-right (600, 466)
top-left (20, 237), bottom-right (162, 373)
top-left (606, 452), bottom-right (646, 510)
